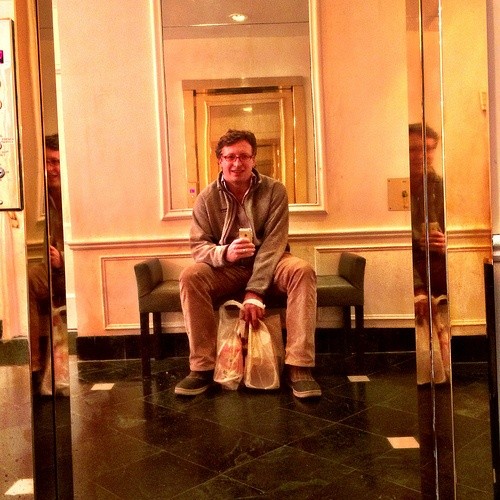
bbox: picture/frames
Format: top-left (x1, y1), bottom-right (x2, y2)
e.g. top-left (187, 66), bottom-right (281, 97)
top-left (0, 17), bottom-right (24, 211)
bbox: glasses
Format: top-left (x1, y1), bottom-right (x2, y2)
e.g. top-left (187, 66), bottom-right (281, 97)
top-left (45, 159), bottom-right (60, 166)
top-left (218, 153), bottom-right (254, 163)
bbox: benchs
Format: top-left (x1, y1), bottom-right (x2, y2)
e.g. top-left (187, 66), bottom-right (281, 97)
top-left (134, 251), bottom-right (366, 379)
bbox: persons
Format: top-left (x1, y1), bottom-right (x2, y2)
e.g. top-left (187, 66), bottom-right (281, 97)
top-left (26, 134), bottom-right (69, 400)
top-left (408, 122), bottom-right (451, 380)
top-left (174, 129), bottom-right (322, 398)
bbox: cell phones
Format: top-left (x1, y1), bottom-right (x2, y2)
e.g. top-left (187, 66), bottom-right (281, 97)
top-left (238, 228), bottom-right (253, 242)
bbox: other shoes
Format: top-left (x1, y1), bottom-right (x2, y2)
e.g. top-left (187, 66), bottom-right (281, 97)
top-left (287, 376), bottom-right (322, 398)
top-left (174, 370), bottom-right (214, 395)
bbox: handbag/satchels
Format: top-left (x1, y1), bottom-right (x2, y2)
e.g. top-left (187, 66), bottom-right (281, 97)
top-left (213, 299), bottom-right (287, 391)
top-left (37, 304), bottom-right (69, 398)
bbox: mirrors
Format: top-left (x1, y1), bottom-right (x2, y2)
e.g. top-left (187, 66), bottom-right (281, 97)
top-left (149, 0), bottom-right (327, 221)
